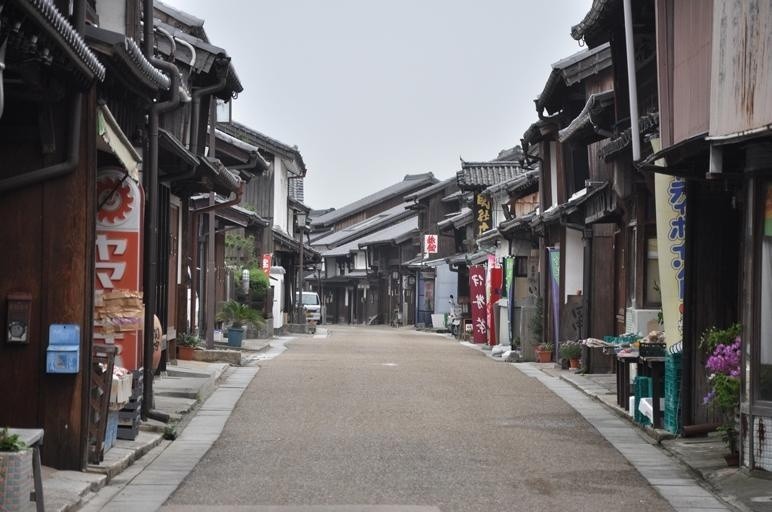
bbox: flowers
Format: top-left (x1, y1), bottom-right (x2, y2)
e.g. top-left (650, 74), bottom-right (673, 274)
top-left (692, 321), bottom-right (741, 457)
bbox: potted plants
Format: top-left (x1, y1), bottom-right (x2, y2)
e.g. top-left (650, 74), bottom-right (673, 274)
top-left (534, 341), bottom-right (582, 368)
top-left (177, 333), bottom-right (200, 359)
top-left (214, 297), bottom-right (267, 347)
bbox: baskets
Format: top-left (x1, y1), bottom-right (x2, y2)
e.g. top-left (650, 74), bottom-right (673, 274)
top-left (639, 343), bottom-right (666, 357)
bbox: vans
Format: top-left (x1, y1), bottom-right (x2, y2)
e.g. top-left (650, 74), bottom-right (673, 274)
top-left (295, 291), bottom-right (321, 324)
